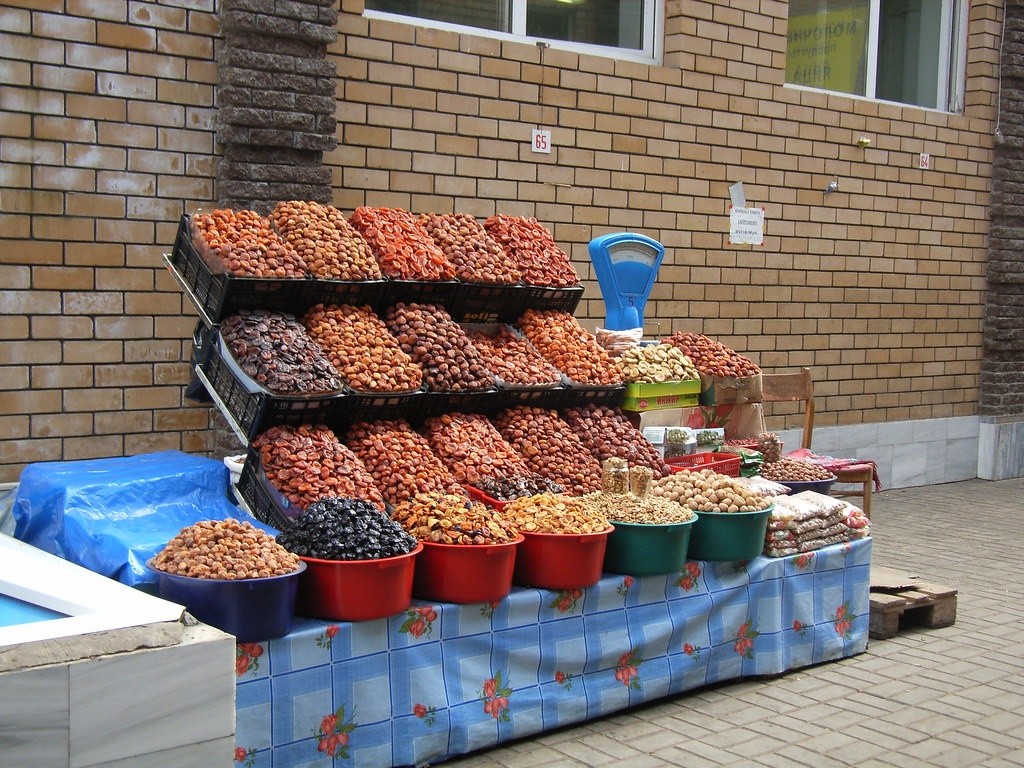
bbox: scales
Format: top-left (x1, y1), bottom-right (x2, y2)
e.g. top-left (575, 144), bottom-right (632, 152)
top-left (588, 233), bottom-right (666, 348)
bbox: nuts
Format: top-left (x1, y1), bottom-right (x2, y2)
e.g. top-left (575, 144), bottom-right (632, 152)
top-left (754, 432), bottom-right (833, 481)
top-left (650, 468), bottom-right (771, 512)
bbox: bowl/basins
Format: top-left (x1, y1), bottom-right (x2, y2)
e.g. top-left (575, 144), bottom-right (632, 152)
top-left (692, 504), bottom-right (775, 561)
top-left (296, 541), bottom-right (423, 620)
top-left (520, 525), bottom-right (617, 589)
top-left (609, 514), bottom-right (700, 577)
top-left (775, 473), bottom-right (838, 496)
top-left (419, 535), bottom-right (525, 603)
top-left (147, 555), bottom-right (307, 643)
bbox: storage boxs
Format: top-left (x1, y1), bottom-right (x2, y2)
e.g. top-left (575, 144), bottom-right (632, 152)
top-left (144, 211), bottom-right (766, 534)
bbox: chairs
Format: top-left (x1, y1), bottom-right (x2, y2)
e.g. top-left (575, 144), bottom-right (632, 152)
top-left (761, 368), bottom-right (874, 522)
